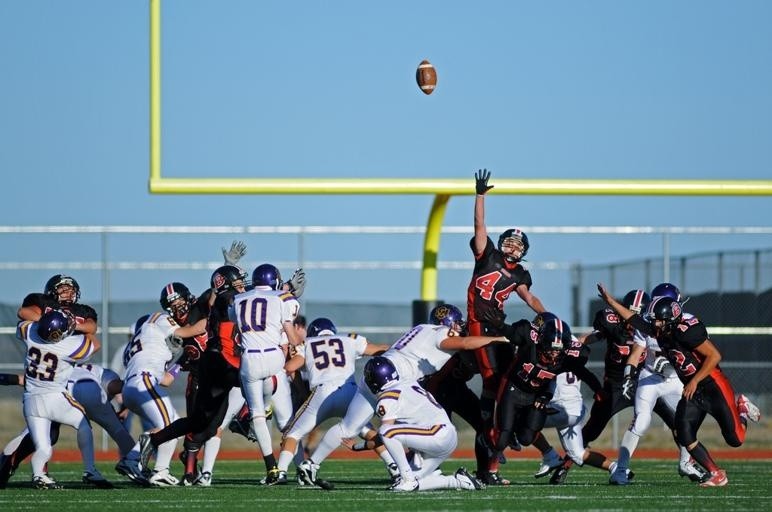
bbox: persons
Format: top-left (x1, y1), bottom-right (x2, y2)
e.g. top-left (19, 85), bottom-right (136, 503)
top-left (0, 275), bottom-right (152, 487)
top-left (122, 239), bottom-right (403, 488)
top-left (298, 168), bottom-right (761, 488)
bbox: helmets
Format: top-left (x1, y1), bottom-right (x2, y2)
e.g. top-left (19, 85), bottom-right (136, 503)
top-left (44, 274), bottom-right (80, 304)
top-left (135, 315), bottom-right (150, 332)
top-left (530, 312), bottom-right (572, 370)
top-left (251, 263), bottom-right (283, 290)
top-left (160, 282), bottom-right (195, 318)
top-left (499, 227), bottom-right (529, 264)
top-left (37, 307), bottom-right (76, 342)
top-left (624, 284), bottom-right (684, 336)
top-left (307, 317), bottom-right (337, 336)
top-left (210, 265), bottom-right (249, 293)
top-left (431, 304), bottom-right (465, 331)
top-left (364, 356), bottom-right (399, 393)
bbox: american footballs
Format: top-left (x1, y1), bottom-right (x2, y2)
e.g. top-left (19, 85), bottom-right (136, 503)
top-left (416, 59), bottom-right (438, 93)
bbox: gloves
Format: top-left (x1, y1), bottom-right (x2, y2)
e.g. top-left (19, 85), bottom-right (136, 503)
top-left (288, 268), bottom-right (306, 298)
top-left (484, 306), bottom-right (506, 331)
top-left (221, 240), bottom-right (247, 264)
top-left (622, 365), bottom-right (636, 401)
top-left (474, 169), bottom-right (494, 194)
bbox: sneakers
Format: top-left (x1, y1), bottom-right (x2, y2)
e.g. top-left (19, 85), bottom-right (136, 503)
top-left (609, 473), bottom-right (627, 485)
top-left (473, 423), bottom-right (521, 463)
top-left (259, 459), bottom-right (320, 486)
top-left (482, 470), bottom-right (510, 485)
top-left (387, 476), bottom-right (419, 493)
top-left (33, 475), bottom-right (64, 489)
top-left (82, 470), bottom-right (112, 487)
top-left (702, 470), bottom-right (728, 487)
top-left (238, 402), bottom-right (272, 423)
top-left (228, 417), bottom-right (259, 442)
top-left (115, 434), bottom-right (213, 487)
top-left (456, 466), bottom-right (486, 490)
top-left (737, 393), bottom-right (760, 421)
top-left (679, 460), bottom-right (709, 482)
top-left (534, 451), bottom-right (565, 478)
top-left (549, 464), bottom-right (568, 484)
top-left (612, 466), bottom-right (634, 480)
